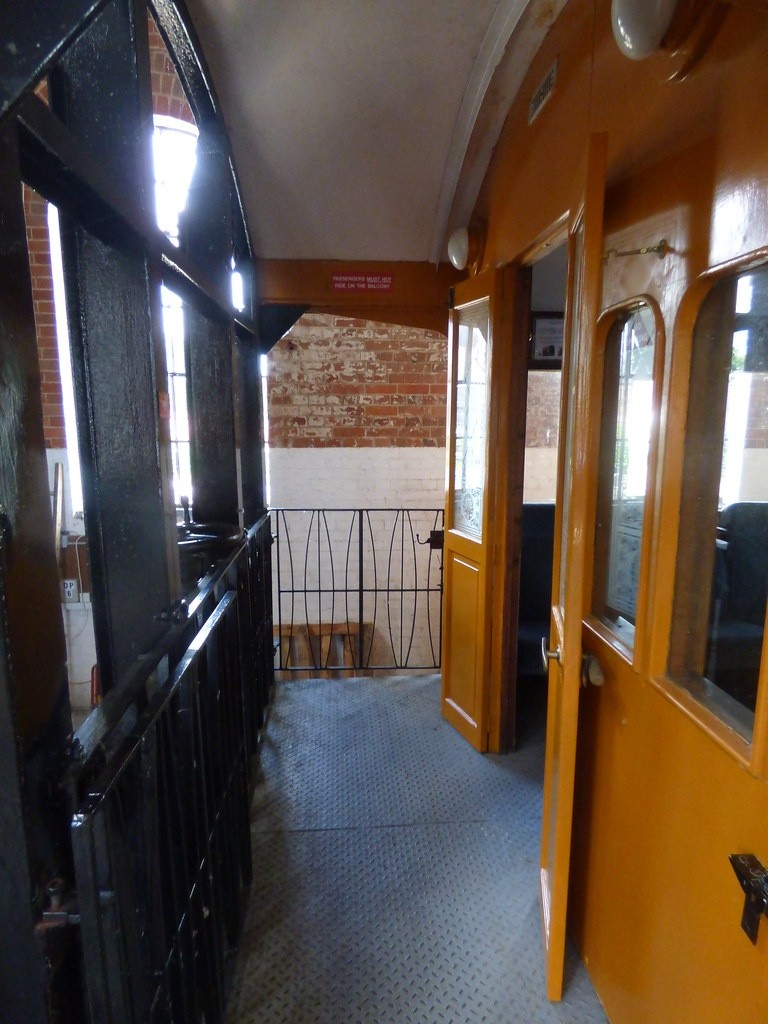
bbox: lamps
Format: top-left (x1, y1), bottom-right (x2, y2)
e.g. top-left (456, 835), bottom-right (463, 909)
top-left (610, 0), bottom-right (725, 81)
top-left (446, 218), bottom-right (485, 271)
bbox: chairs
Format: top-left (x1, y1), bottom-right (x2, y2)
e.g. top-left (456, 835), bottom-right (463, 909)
top-left (705, 503), bottom-right (767, 687)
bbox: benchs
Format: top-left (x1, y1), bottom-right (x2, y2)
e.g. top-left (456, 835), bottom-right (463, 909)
top-left (271, 622), bottom-right (374, 678)
